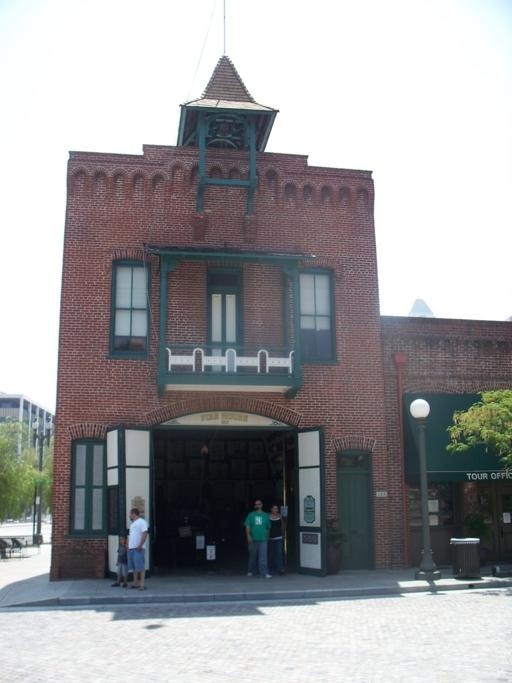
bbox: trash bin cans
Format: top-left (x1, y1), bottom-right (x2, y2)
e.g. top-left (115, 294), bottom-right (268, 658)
top-left (450, 538), bottom-right (481, 578)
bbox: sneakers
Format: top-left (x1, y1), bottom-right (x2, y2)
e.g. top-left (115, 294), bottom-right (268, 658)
top-left (265, 575), bottom-right (272, 579)
top-left (280, 572), bottom-right (284, 576)
top-left (111, 583), bottom-right (146, 591)
top-left (247, 572), bottom-right (252, 576)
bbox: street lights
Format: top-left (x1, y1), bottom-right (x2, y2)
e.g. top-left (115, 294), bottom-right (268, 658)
top-left (32, 417), bottom-right (52, 545)
top-left (409, 398), bottom-right (441, 580)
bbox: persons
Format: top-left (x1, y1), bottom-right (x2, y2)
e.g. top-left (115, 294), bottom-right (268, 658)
top-left (242, 498), bottom-right (273, 579)
top-left (124, 507), bottom-right (149, 590)
top-left (111, 532), bottom-right (129, 587)
top-left (262, 503), bottom-right (287, 575)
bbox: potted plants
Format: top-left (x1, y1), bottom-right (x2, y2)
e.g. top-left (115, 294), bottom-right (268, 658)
top-left (326, 517), bottom-right (348, 575)
